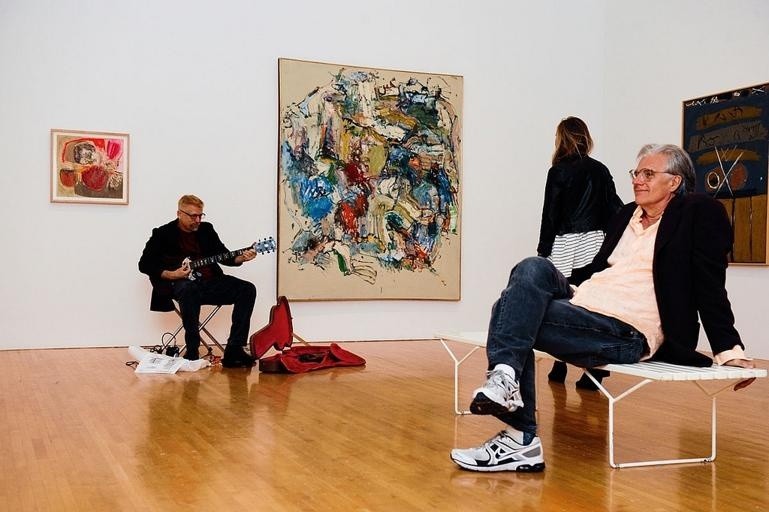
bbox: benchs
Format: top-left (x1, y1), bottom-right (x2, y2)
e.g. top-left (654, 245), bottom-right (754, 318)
top-left (432, 322), bottom-right (769, 469)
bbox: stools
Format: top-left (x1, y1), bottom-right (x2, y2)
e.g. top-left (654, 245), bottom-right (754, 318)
top-left (150, 288), bottom-right (225, 363)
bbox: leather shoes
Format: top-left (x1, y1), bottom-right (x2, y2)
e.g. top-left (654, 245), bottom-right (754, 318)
top-left (223, 347), bottom-right (254, 367)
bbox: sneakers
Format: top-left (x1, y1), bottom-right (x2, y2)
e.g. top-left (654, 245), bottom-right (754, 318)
top-left (470, 370), bottom-right (524, 415)
top-left (450, 430), bottom-right (545, 472)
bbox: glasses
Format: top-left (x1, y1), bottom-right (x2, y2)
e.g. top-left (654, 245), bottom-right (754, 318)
top-left (179, 209), bottom-right (205, 218)
top-left (630, 168), bottom-right (668, 179)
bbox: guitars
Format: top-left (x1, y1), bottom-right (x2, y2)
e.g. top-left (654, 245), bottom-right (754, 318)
top-left (162, 236), bottom-right (277, 299)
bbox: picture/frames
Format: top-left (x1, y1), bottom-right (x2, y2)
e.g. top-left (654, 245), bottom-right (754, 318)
top-left (276, 56), bottom-right (462, 302)
top-left (678, 80), bottom-right (769, 267)
top-left (50, 128), bottom-right (130, 206)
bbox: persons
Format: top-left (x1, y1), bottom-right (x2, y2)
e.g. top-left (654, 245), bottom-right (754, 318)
top-left (534, 117), bottom-right (621, 389)
top-left (139, 198), bottom-right (256, 372)
top-left (445, 144), bottom-right (758, 478)
